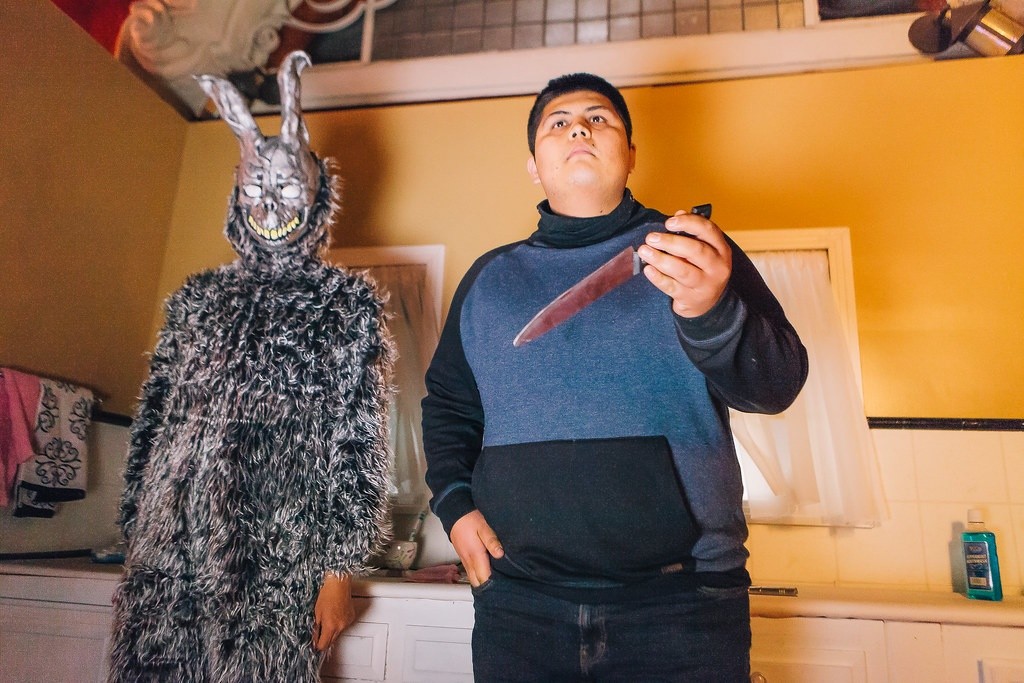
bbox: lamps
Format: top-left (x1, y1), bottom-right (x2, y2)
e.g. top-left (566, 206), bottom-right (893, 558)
top-left (907, 1), bottom-right (1024, 56)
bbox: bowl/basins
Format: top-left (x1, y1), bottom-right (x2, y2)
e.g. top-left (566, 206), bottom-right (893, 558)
top-left (376, 541), bottom-right (417, 569)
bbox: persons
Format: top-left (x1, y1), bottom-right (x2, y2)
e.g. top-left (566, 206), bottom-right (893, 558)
top-left (422, 73), bottom-right (809, 683)
top-left (105, 50), bottom-right (399, 683)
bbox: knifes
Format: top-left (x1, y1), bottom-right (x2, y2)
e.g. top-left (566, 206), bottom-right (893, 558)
top-left (513, 203), bottom-right (713, 351)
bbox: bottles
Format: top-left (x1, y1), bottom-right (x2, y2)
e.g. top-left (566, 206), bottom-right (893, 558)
top-left (961, 508), bottom-right (1004, 601)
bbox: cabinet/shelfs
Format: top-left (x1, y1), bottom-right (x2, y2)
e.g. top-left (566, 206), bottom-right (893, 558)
top-left (0, 574), bottom-right (1024, 683)
